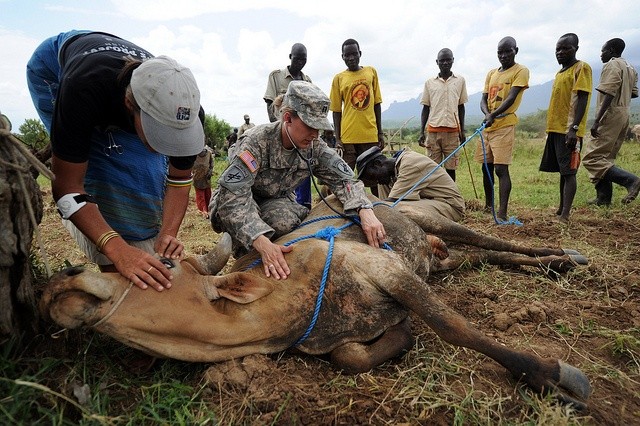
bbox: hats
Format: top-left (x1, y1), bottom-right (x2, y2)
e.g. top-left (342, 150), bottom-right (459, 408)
top-left (280, 80), bottom-right (335, 131)
top-left (130, 54), bottom-right (205, 157)
top-left (356, 145), bottom-right (383, 180)
top-left (244, 115), bottom-right (250, 120)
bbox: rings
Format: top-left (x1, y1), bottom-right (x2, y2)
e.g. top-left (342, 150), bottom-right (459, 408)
top-left (146, 266), bottom-right (154, 272)
top-left (377, 230), bottom-right (383, 234)
top-left (268, 265), bottom-right (274, 268)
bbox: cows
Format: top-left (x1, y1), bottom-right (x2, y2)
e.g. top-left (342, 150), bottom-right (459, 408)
top-left (37, 190), bottom-right (591, 412)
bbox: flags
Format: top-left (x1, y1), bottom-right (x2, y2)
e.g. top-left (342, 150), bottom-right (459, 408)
top-left (238, 149), bottom-right (260, 173)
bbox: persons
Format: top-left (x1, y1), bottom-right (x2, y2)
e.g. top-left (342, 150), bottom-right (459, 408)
top-left (237, 114), bottom-right (255, 141)
top-left (538, 31), bottom-right (592, 223)
top-left (262, 42), bottom-right (312, 214)
top-left (355, 145), bottom-right (466, 222)
top-left (226, 126), bottom-right (238, 148)
top-left (192, 148), bottom-right (215, 219)
top-left (26, 29), bottom-right (206, 293)
top-left (319, 130), bottom-right (337, 148)
top-left (329, 38), bottom-right (385, 198)
top-left (417, 48), bottom-right (469, 184)
top-left (472, 35), bottom-right (530, 223)
top-left (580, 38), bottom-right (639, 205)
top-left (207, 80), bottom-right (387, 280)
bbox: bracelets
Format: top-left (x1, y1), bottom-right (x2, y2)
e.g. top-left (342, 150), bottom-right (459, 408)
top-left (95, 231), bottom-right (120, 255)
top-left (570, 124), bottom-right (579, 129)
top-left (165, 174), bottom-right (194, 187)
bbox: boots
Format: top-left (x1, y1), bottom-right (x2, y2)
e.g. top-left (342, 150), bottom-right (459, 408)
top-left (588, 179), bottom-right (612, 205)
top-left (604, 165), bottom-right (640, 204)
